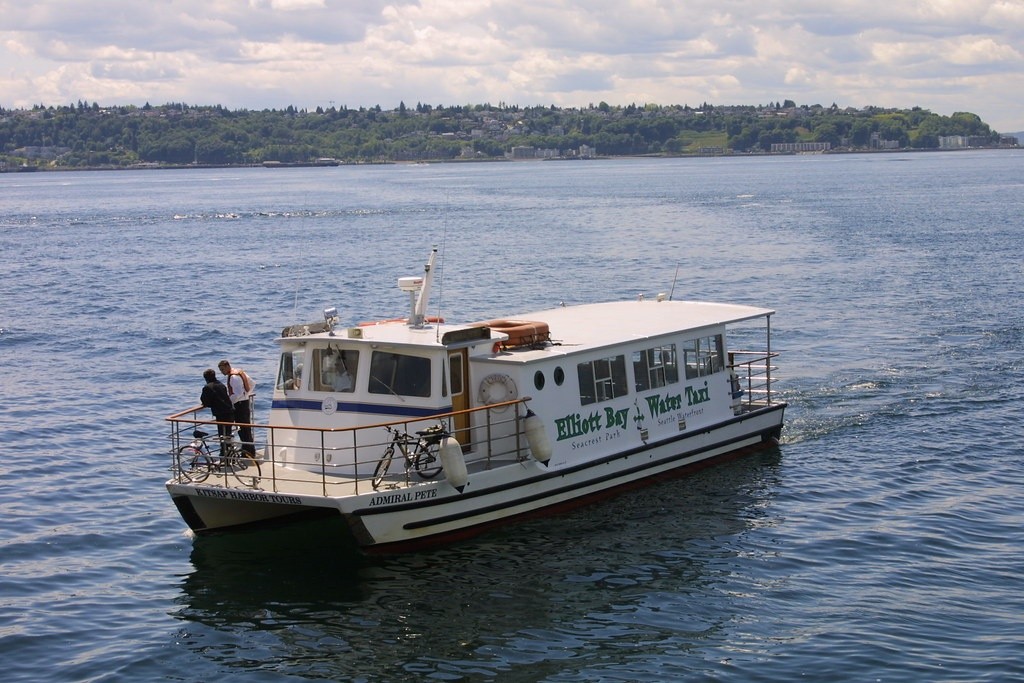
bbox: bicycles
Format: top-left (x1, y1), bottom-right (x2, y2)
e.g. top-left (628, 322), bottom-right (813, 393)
top-left (177, 425), bottom-right (262, 488)
top-left (370, 420), bottom-right (452, 487)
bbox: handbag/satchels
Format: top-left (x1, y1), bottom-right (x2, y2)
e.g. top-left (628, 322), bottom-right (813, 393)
top-left (422, 423), bottom-right (446, 441)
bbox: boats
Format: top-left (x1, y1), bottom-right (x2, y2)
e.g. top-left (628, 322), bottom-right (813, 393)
top-left (161, 191), bottom-right (790, 559)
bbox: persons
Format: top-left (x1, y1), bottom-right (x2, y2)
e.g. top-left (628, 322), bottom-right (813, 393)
top-left (330, 355), bottom-right (356, 393)
top-left (218, 360), bottom-right (256, 458)
top-left (200, 369), bottom-right (236, 465)
top-left (654, 350), bottom-right (674, 377)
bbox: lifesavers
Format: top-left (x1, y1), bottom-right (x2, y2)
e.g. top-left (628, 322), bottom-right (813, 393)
top-left (480, 372), bottom-right (518, 409)
top-left (358, 316), bottom-right (445, 327)
top-left (467, 319), bottom-right (549, 345)
